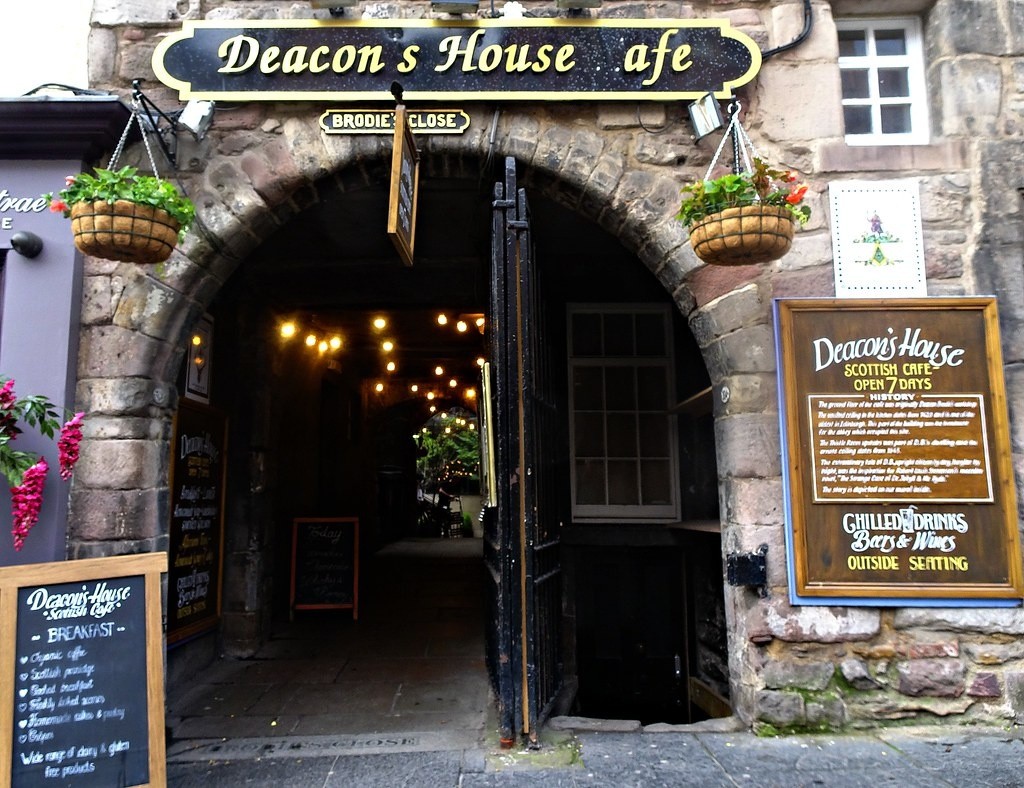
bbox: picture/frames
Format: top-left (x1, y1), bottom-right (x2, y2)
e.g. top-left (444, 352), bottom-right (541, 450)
top-left (184, 312), bottom-right (214, 405)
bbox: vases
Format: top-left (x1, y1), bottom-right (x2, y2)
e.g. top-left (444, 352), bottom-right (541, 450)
top-left (68, 200), bottom-right (184, 263)
top-left (691, 206), bottom-right (793, 265)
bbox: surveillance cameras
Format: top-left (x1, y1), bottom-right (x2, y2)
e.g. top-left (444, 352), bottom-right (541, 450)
top-left (11, 232), bottom-right (43, 259)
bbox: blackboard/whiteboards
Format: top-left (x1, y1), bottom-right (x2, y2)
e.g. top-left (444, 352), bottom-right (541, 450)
top-left (168, 395), bottom-right (228, 643)
top-left (288, 516), bottom-right (360, 610)
top-left (387, 104), bottom-right (421, 264)
top-left (0, 552), bottom-right (171, 788)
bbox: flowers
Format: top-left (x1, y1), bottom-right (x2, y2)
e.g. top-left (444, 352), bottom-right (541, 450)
top-left (51, 167), bottom-right (193, 232)
top-left (0, 378), bottom-right (83, 551)
top-left (678, 159), bottom-right (811, 230)
